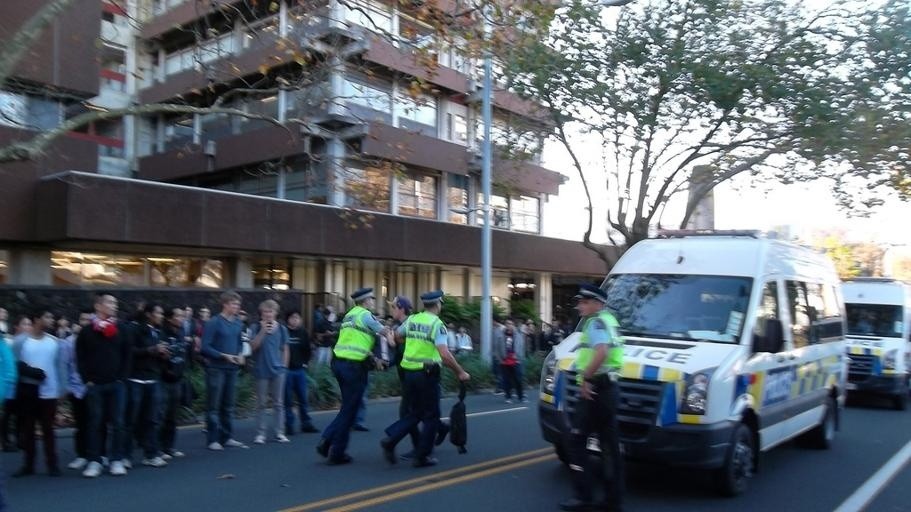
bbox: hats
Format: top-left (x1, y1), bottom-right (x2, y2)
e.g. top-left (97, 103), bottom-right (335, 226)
top-left (386, 295), bottom-right (411, 312)
top-left (420, 290), bottom-right (445, 304)
top-left (573, 283), bottom-right (608, 304)
top-left (351, 288), bottom-right (376, 302)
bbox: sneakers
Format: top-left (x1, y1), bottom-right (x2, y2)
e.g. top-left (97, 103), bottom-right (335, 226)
top-left (434, 423), bottom-right (450, 445)
top-left (222, 438), bottom-right (243, 446)
top-left (400, 448), bottom-right (417, 461)
top-left (82, 460), bottom-right (104, 477)
top-left (206, 441), bottom-right (224, 451)
top-left (559, 496), bottom-right (593, 511)
top-left (254, 435), bottom-right (266, 444)
top-left (156, 451), bottom-right (172, 459)
top-left (141, 457), bottom-right (167, 467)
top-left (271, 433), bottom-right (290, 443)
top-left (67, 457), bottom-right (88, 470)
top-left (110, 461), bottom-right (127, 475)
top-left (589, 500), bottom-right (624, 512)
top-left (47, 463), bottom-right (60, 476)
top-left (163, 448), bottom-right (184, 456)
top-left (301, 425), bottom-right (320, 433)
top-left (121, 458), bottom-right (133, 468)
top-left (382, 437), bottom-right (399, 465)
top-left (505, 398), bottom-right (528, 404)
top-left (101, 456), bottom-right (109, 466)
top-left (353, 425), bottom-right (368, 431)
top-left (286, 428), bottom-right (294, 435)
top-left (414, 456), bottom-right (438, 466)
top-left (10, 467), bottom-right (34, 478)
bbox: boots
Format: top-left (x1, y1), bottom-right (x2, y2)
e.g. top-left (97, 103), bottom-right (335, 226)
top-left (327, 446), bottom-right (353, 464)
top-left (317, 438), bottom-right (332, 458)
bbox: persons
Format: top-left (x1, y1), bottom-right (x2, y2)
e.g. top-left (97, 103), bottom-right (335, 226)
top-left (355, 310), bottom-right (381, 434)
top-left (1, 286), bottom-right (320, 480)
top-left (381, 295), bottom-right (452, 457)
top-left (502, 317), bottom-right (531, 405)
top-left (315, 302), bottom-right (571, 369)
top-left (379, 290), bottom-right (472, 469)
top-left (491, 313), bottom-right (510, 396)
top-left (559, 283), bottom-right (625, 512)
top-left (313, 287), bottom-right (391, 464)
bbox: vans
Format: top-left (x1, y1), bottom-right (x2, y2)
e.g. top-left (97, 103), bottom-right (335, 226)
top-left (539, 225), bottom-right (852, 498)
top-left (840, 278), bottom-right (910, 411)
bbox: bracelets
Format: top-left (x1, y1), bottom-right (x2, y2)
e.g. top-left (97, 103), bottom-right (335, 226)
top-left (584, 376), bottom-right (594, 383)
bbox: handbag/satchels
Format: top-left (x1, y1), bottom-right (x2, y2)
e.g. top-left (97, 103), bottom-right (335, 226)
top-left (181, 378), bottom-right (194, 409)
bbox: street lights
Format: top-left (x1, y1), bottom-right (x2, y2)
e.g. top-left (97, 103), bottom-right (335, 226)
top-left (477, 0), bottom-right (635, 376)
